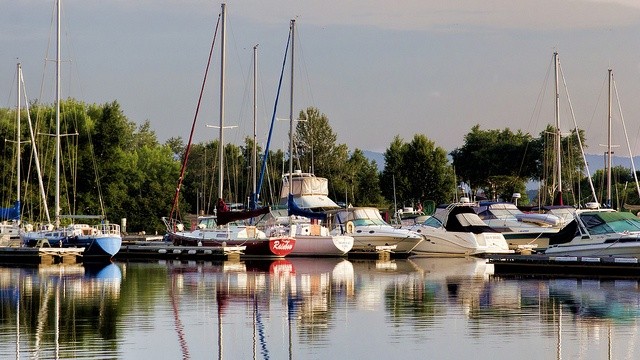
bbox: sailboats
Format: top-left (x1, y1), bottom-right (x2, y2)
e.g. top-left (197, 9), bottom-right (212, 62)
top-left (0, 57), bottom-right (54, 238)
top-left (162, 3), bottom-right (296, 258)
top-left (19, 0), bottom-right (122, 259)
top-left (249, 20), bottom-right (354, 256)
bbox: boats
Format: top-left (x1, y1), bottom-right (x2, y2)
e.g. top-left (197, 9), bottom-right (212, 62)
top-left (434, 167), bottom-right (562, 233)
top-left (392, 202), bottom-right (515, 255)
top-left (197, 170), bottom-right (424, 259)
top-left (531, 212), bottom-right (640, 258)
top-left (509, 51), bottom-right (616, 223)
